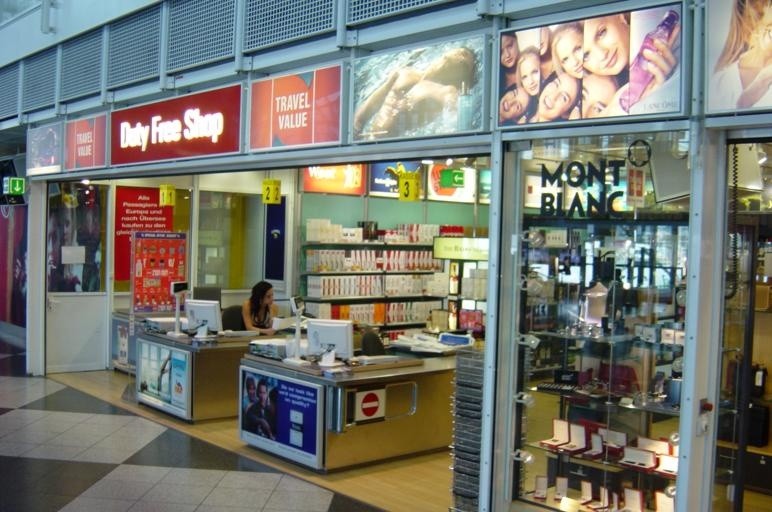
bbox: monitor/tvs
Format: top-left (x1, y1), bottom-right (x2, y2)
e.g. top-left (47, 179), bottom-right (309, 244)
top-left (306, 318), bottom-right (354, 367)
top-left (184, 299), bottom-right (223, 338)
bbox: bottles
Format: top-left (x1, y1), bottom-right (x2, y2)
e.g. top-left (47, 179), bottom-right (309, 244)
top-left (177, 260), bottom-right (184, 278)
top-left (135, 259), bottom-right (143, 278)
top-left (629, 9), bottom-right (679, 107)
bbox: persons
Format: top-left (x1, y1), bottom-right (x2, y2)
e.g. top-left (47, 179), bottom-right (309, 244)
top-left (242, 377), bottom-right (259, 425)
top-left (501, 80), bottom-right (532, 129)
top-left (47, 216), bottom-right (80, 290)
top-left (267, 387), bottom-right (277, 422)
top-left (15, 249), bottom-right (33, 308)
top-left (517, 41), bottom-right (542, 96)
top-left (527, 11), bottom-right (681, 125)
top-left (708, 1), bottom-right (772, 111)
top-left (240, 281), bottom-right (279, 336)
top-left (499, 34), bottom-right (523, 84)
top-left (61, 199), bottom-right (83, 292)
top-left (248, 378), bottom-right (275, 439)
top-left (78, 205), bottom-right (103, 293)
top-left (353, 47), bottom-right (477, 141)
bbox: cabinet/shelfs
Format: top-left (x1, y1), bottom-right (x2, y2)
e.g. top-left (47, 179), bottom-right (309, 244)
top-left (298, 238), bottom-right (445, 331)
top-left (516, 208), bottom-right (771, 512)
